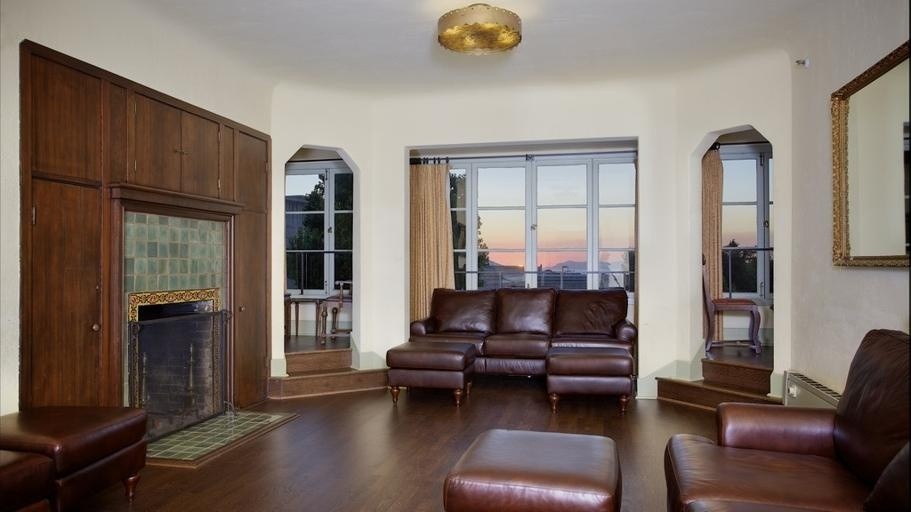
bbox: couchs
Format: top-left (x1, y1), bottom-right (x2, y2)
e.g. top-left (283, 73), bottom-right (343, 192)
top-left (410, 286), bottom-right (638, 399)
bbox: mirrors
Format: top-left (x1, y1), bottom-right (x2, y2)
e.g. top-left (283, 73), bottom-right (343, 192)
top-left (830, 40), bottom-right (911, 268)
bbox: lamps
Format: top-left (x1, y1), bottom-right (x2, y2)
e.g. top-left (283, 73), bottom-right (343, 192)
top-left (437, 3), bottom-right (522, 56)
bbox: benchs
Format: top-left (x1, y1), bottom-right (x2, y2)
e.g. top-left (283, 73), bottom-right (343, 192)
top-left (386, 342), bottom-right (475, 408)
top-left (0, 405), bottom-right (149, 512)
top-left (443, 428), bottom-right (622, 512)
top-left (545, 348), bottom-right (635, 416)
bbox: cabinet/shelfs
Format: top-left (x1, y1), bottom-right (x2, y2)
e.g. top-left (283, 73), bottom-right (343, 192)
top-left (131, 91), bottom-right (220, 197)
top-left (235, 127), bottom-right (269, 405)
top-left (31, 55), bottom-right (106, 408)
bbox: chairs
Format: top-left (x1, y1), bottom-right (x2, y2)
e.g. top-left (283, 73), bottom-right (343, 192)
top-left (702, 253), bottom-right (762, 354)
top-left (663, 329), bottom-right (911, 512)
